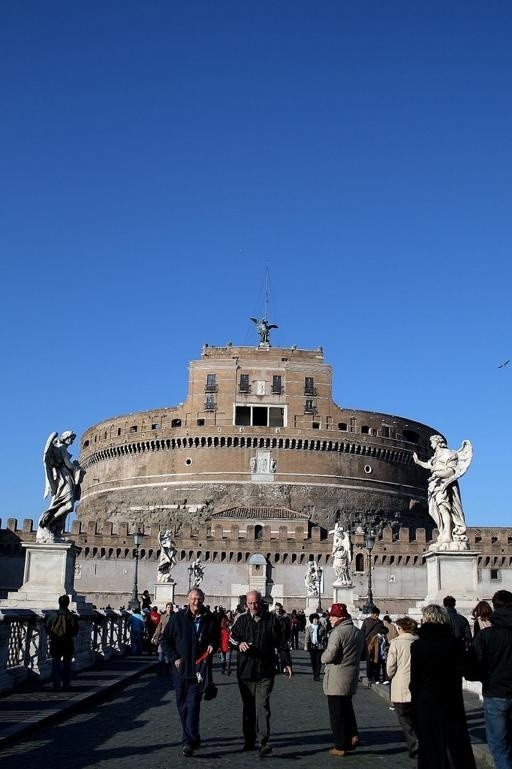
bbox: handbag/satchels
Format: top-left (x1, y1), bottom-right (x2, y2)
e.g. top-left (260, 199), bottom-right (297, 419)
top-left (204, 653), bottom-right (217, 701)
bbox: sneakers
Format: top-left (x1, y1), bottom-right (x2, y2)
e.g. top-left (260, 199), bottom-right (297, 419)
top-left (374, 680), bottom-right (380, 684)
top-left (181, 738), bottom-right (200, 756)
top-left (257, 745), bottom-right (272, 757)
top-left (351, 735), bottom-right (359, 750)
top-left (409, 739), bottom-right (419, 758)
top-left (382, 680), bottom-right (390, 685)
top-left (328, 747), bottom-right (344, 756)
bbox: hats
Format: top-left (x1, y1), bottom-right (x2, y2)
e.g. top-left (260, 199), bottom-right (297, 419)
top-left (327, 603), bottom-right (348, 616)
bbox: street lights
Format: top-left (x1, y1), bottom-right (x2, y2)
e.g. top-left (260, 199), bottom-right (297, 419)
top-left (187, 565), bottom-right (194, 591)
top-left (316, 566), bottom-right (326, 613)
top-left (361, 529), bottom-right (381, 616)
top-left (128, 526), bottom-right (149, 611)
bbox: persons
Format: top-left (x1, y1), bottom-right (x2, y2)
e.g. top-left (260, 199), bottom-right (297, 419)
top-left (303, 561), bottom-right (319, 594)
top-left (385, 615), bottom-right (419, 759)
top-left (229, 589), bottom-right (294, 757)
top-left (464, 589), bottom-right (511, 769)
top-left (192, 559), bottom-right (205, 587)
top-left (332, 527), bottom-right (350, 581)
top-left (206, 605), bottom-right (247, 674)
top-left (412, 434), bottom-right (473, 542)
top-left (410, 604), bottom-right (477, 768)
top-left (150, 602), bottom-right (188, 677)
top-left (156, 528), bottom-right (177, 574)
top-left (38, 431), bottom-right (85, 528)
top-left (272, 604), bottom-right (333, 681)
top-left (321, 603), bottom-right (369, 757)
top-left (44, 594), bottom-right (80, 690)
top-left (159, 589), bottom-right (220, 758)
top-left (443, 595), bottom-right (492, 647)
top-left (107, 589), bottom-right (161, 656)
top-left (361, 610), bottom-right (398, 684)
